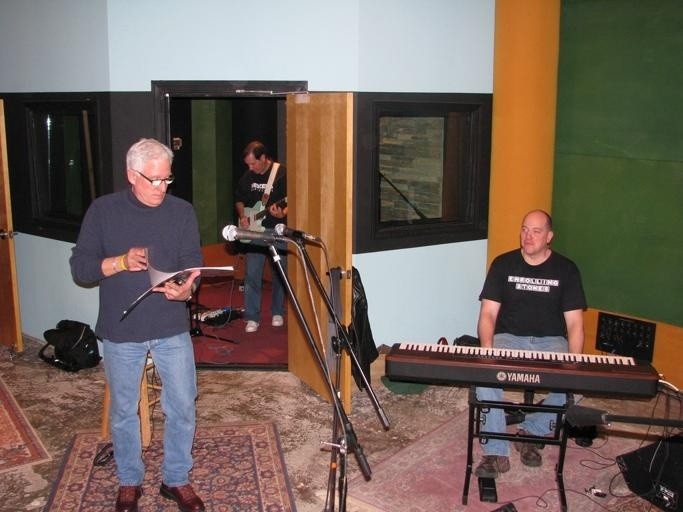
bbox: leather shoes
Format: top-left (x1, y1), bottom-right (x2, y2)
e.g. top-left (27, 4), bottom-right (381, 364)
top-left (160, 480), bottom-right (204, 512)
top-left (475, 456), bottom-right (509, 478)
top-left (515, 430), bottom-right (541, 466)
top-left (116, 486), bottom-right (143, 512)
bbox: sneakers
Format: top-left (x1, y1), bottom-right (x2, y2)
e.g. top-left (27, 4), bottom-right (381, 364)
top-left (272, 315), bottom-right (283, 327)
top-left (245, 320), bottom-right (260, 332)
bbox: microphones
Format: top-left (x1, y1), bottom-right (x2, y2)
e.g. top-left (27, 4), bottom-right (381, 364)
top-left (274, 222), bottom-right (323, 245)
top-left (222, 224), bottom-right (289, 244)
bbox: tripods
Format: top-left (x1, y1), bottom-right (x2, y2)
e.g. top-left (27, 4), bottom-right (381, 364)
top-left (189, 288), bottom-right (242, 345)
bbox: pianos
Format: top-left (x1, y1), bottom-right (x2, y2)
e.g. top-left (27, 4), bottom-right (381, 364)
top-left (383, 341), bottom-right (660, 404)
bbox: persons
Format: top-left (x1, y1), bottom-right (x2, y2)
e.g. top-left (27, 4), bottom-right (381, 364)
top-left (69, 139), bottom-right (207, 512)
top-left (234, 141), bottom-right (287, 332)
top-left (474, 210), bottom-right (586, 480)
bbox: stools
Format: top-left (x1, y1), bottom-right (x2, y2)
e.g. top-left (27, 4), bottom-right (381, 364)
top-left (453, 336), bottom-right (540, 417)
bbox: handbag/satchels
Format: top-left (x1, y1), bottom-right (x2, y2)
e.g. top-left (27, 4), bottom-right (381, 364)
top-left (39, 320), bottom-right (102, 372)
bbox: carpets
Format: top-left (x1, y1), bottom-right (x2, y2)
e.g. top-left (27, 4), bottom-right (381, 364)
top-left (0, 376), bottom-right (54, 472)
top-left (44, 418), bottom-right (297, 512)
top-left (342, 397), bottom-right (660, 510)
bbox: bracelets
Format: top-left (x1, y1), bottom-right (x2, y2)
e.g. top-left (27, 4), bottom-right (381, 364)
top-left (185, 289), bottom-right (193, 303)
top-left (118, 254), bottom-right (128, 271)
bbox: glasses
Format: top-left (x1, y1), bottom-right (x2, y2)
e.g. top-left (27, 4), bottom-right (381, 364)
top-left (136, 171), bottom-right (174, 186)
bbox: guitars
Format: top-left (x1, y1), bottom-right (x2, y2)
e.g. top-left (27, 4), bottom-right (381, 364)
top-left (236, 195), bottom-right (287, 245)
